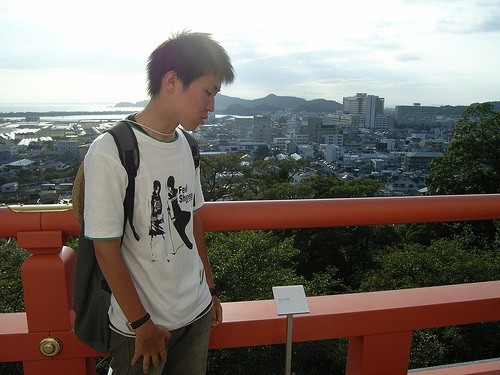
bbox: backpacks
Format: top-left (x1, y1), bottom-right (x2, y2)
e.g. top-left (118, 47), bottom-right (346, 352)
top-left (72, 121), bottom-right (201, 354)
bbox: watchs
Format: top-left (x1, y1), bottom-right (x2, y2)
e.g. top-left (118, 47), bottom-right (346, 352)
top-left (126, 311), bottom-right (151, 332)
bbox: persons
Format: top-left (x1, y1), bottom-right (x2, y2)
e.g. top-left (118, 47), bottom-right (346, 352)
top-left (81, 29), bottom-right (236, 375)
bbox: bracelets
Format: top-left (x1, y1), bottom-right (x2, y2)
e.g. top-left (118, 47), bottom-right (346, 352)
top-left (209, 288), bottom-right (218, 295)
